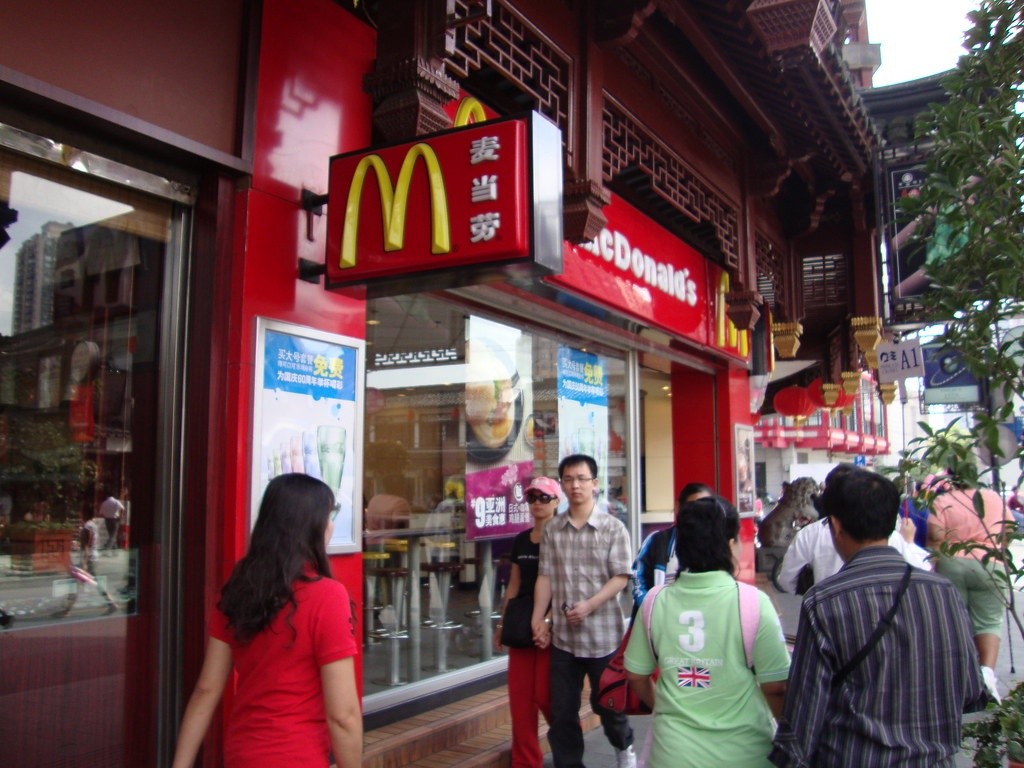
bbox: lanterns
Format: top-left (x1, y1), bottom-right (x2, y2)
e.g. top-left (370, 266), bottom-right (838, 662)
top-left (773, 384), bottom-right (816, 429)
top-left (807, 376), bottom-right (855, 417)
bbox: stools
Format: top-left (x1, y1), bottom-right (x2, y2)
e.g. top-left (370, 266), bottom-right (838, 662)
top-left (357, 538), bottom-right (504, 688)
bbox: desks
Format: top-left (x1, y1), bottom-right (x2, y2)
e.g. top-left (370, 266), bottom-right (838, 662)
top-left (368, 526), bottom-right (495, 684)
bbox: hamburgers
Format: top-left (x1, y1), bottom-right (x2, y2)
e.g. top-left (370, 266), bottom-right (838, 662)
top-left (464, 364), bottom-right (516, 448)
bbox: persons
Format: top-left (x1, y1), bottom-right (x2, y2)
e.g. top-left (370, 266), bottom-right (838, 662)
top-left (363, 471), bottom-right (412, 630)
top-left (491, 455), bottom-right (1024, 768)
top-left (172, 472), bottom-right (363, 768)
top-left (424, 477), bottom-right (468, 563)
top-left (60, 489), bottom-right (125, 616)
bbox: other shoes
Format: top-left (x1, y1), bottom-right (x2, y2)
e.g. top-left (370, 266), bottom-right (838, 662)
top-left (980, 664), bottom-right (1001, 704)
top-left (615, 743), bottom-right (637, 768)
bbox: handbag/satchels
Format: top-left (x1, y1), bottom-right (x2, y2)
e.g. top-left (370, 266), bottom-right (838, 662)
top-left (501, 597), bottom-right (535, 649)
top-left (599, 628), bottom-right (659, 714)
top-left (795, 564), bottom-right (816, 596)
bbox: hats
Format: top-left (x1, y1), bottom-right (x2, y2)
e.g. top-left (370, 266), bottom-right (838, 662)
top-left (523, 476), bottom-right (561, 500)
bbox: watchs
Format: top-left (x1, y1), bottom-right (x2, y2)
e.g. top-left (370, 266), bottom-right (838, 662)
top-left (496, 625), bottom-right (502, 628)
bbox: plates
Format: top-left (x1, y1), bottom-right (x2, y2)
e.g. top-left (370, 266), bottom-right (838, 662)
top-left (464, 337), bottom-right (524, 462)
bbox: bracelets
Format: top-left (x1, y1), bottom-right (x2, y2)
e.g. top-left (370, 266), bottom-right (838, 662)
top-left (543, 617), bottom-right (552, 624)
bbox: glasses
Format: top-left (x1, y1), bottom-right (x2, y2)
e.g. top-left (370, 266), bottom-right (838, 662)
top-left (562, 601), bottom-right (574, 612)
top-left (526, 493), bottom-right (557, 504)
top-left (562, 476), bottom-right (594, 483)
